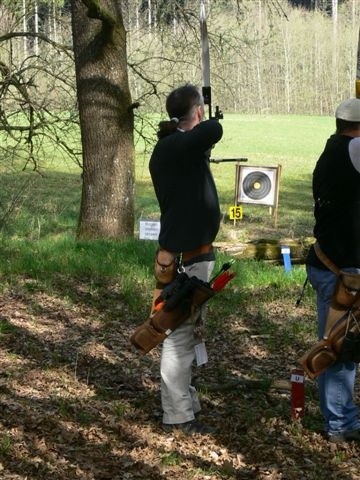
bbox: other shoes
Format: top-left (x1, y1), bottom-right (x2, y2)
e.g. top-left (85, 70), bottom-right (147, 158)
top-left (327, 429), bottom-right (360, 443)
top-left (166, 419), bottom-right (217, 434)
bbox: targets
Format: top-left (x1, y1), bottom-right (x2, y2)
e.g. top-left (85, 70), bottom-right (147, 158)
top-left (237, 165), bottom-right (278, 207)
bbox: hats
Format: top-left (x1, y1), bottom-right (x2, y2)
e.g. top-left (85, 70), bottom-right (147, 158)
top-left (335, 98), bottom-right (360, 122)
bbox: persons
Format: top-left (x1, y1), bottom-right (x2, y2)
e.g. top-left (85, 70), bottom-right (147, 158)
top-left (148, 83), bottom-right (224, 436)
top-left (303, 98), bottom-right (360, 443)
